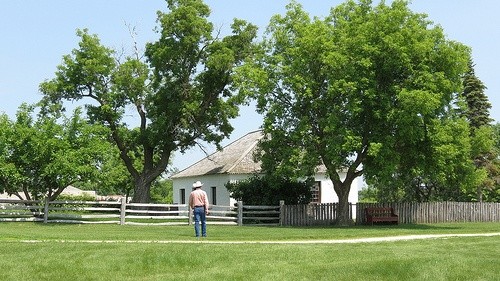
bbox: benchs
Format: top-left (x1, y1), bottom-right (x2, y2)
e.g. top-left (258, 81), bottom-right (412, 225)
top-left (366, 208), bottom-right (398, 224)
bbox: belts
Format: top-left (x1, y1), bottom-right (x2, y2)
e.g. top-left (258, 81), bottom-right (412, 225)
top-left (194, 205), bottom-right (205, 208)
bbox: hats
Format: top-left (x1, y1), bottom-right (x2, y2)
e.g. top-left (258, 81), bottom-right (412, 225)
top-left (193, 181), bottom-right (204, 188)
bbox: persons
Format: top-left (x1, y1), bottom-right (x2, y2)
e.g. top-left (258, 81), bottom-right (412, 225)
top-left (189, 181), bottom-right (209, 237)
